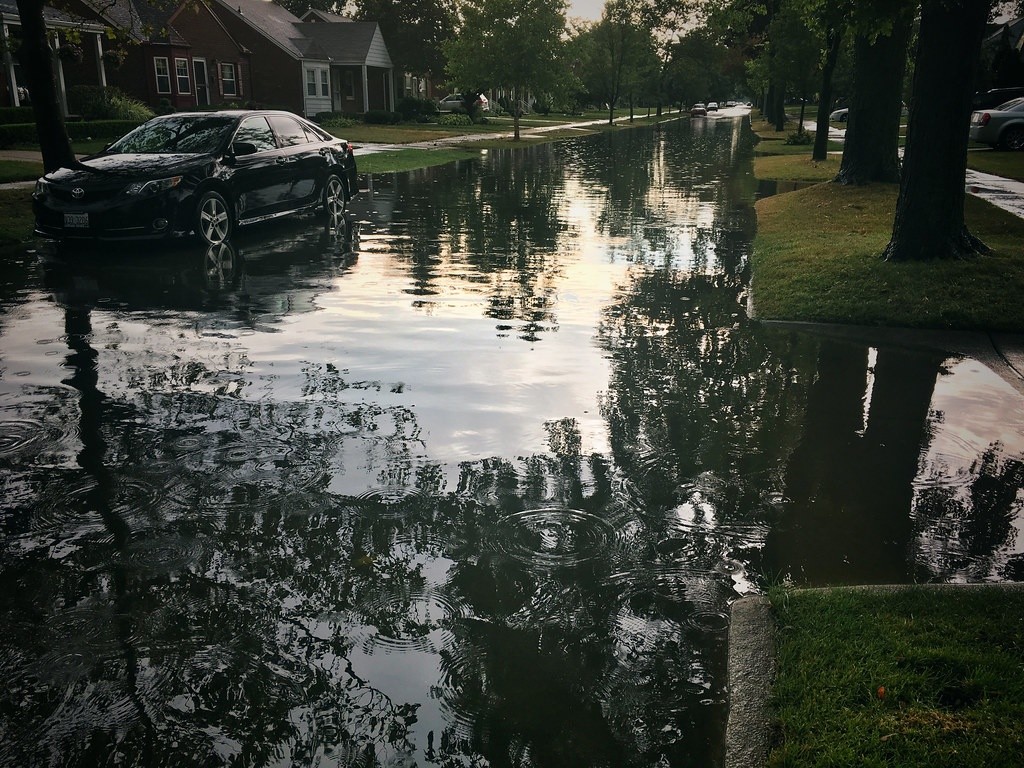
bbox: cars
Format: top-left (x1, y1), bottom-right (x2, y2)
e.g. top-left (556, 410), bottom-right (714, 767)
top-left (690, 103), bottom-right (707, 116)
top-left (438, 92), bottom-right (490, 114)
top-left (707, 102), bottom-right (719, 111)
top-left (726, 100), bottom-right (752, 106)
top-left (31, 108), bottom-right (360, 249)
top-left (829, 100), bottom-right (908, 123)
top-left (969, 97), bottom-right (1024, 153)
